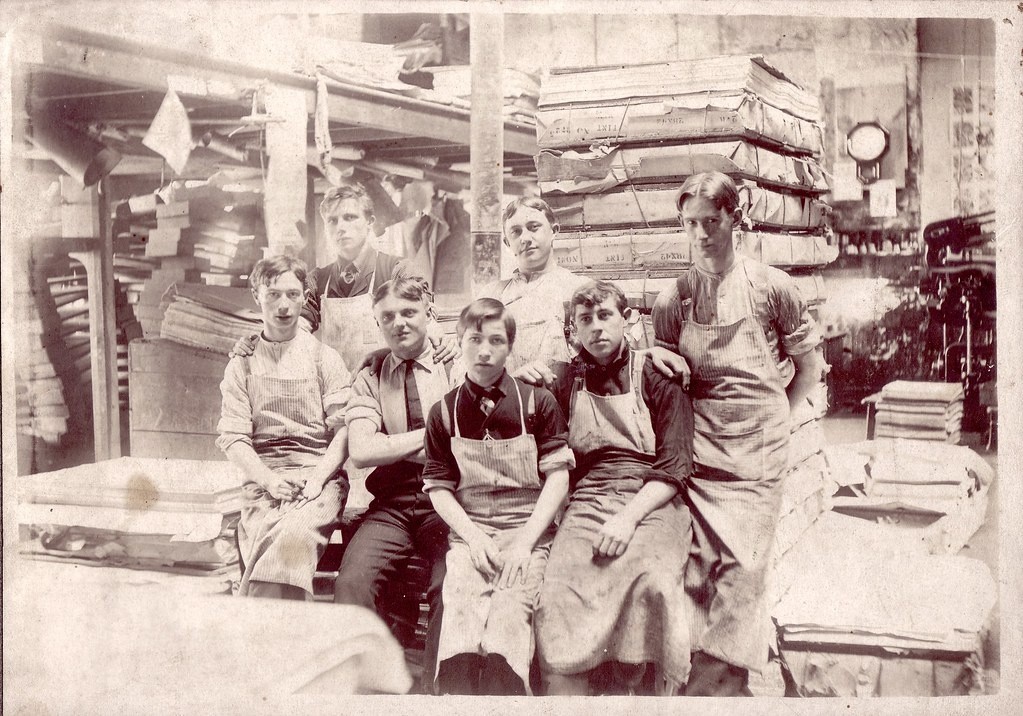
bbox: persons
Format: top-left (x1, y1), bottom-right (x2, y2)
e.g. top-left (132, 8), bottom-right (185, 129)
top-left (227, 182), bottom-right (462, 365)
top-left (215, 254), bottom-right (350, 600)
top-left (652, 169), bottom-right (822, 697)
top-left (335, 199), bottom-right (690, 697)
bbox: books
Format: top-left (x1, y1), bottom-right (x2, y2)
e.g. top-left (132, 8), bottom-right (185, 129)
top-left (874, 379), bottom-right (965, 449)
top-left (45, 198), bottom-right (267, 409)
